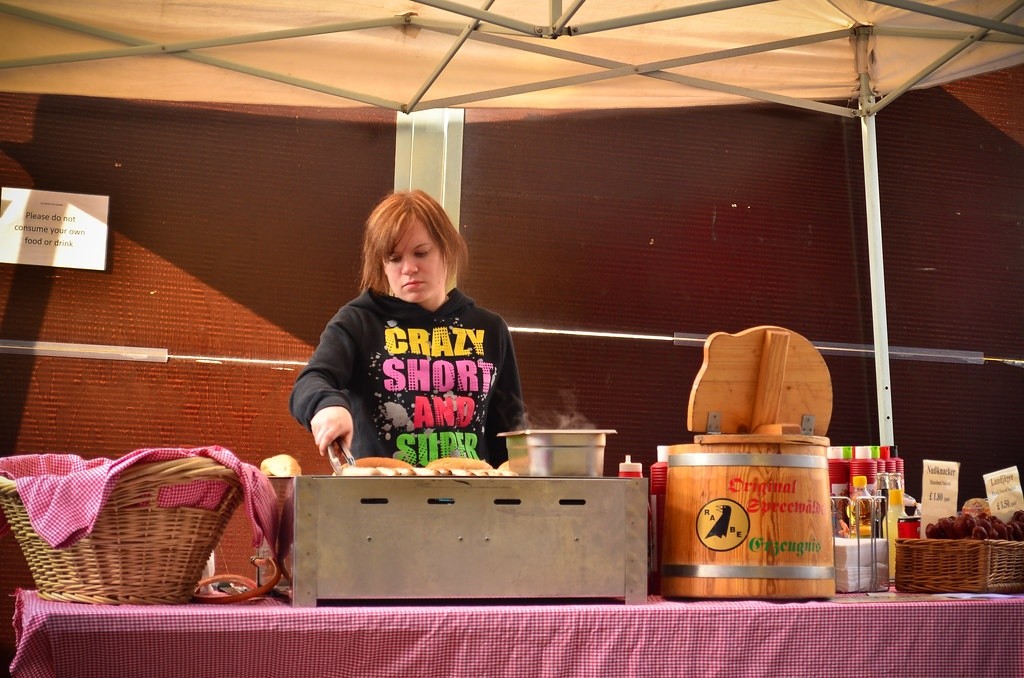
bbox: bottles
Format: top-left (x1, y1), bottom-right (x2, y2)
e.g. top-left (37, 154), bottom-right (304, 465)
top-left (826, 444), bottom-right (905, 582)
top-left (618, 454), bottom-right (644, 478)
top-left (651, 444), bottom-right (671, 594)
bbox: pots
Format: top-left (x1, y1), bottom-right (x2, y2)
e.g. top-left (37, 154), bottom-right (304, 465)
top-left (497, 428), bottom-right (617, 478)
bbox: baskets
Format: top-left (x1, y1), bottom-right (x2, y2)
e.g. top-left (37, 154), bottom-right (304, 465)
top-left (890, 534), bottom-right (1024, 594)
top-left (0, 445), bottom-right (247, 605)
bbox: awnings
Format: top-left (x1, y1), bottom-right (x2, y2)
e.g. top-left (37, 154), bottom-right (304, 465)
top-left (0, 3), bottom-right (1024, 458)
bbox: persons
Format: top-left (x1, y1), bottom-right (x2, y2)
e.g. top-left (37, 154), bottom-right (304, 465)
top-left (286, 188), bottom-right (526, 471)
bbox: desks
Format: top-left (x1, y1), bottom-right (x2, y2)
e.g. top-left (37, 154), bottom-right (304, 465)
top-left (10, 583), bottom-right (1024, 678)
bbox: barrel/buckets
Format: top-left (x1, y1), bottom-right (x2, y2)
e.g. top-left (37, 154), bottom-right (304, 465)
top-left (660, 325), bottom-right (838, 600)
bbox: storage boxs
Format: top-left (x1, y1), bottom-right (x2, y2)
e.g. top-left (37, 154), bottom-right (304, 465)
top-left (1, 446), bottom-right (251, 605)
top-left (653, 433), bottom-right (840, 603)
